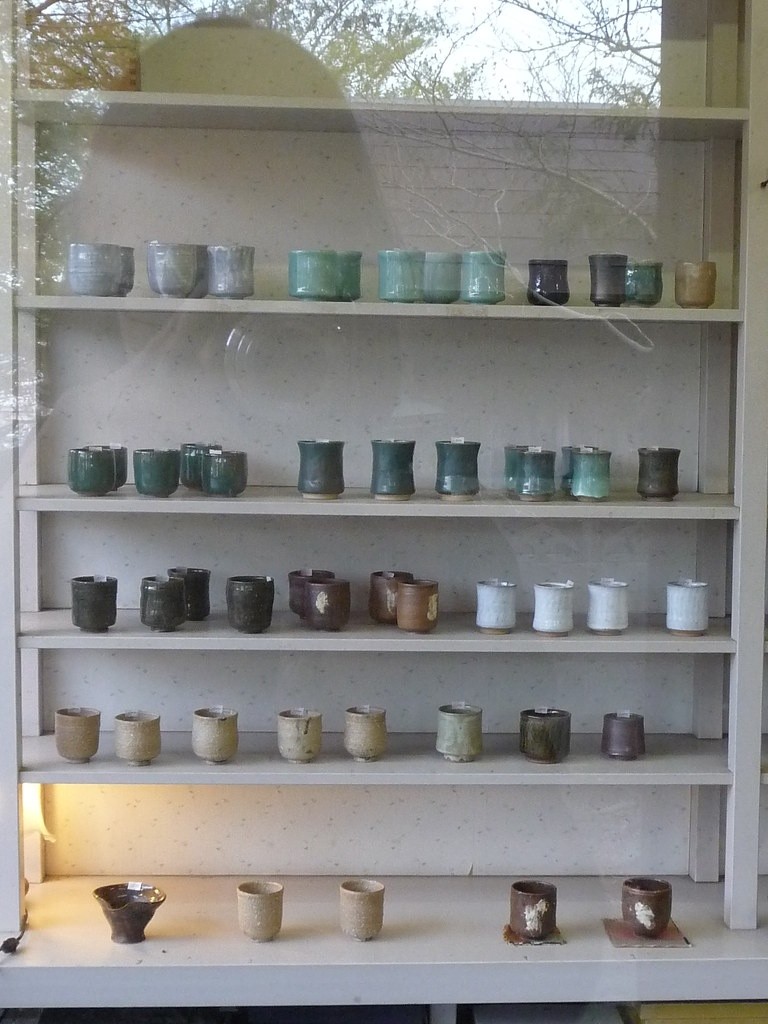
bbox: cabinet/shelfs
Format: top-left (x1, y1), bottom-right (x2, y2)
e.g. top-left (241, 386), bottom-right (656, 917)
top-left (0, 0), bottom-right (768, 1008)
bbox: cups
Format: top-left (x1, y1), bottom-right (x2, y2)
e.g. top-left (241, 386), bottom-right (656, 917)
top-left (68, 438), bottom-right (612, 503)
top-left (676, 260), bottom-right (716, 309)
top-left (67, 242), bottom-right (664, 308)
top-left (226, 576), bottom-right (274, 633)
top-left (287, 569), bottom-right (350, 632)
top-left (141, 567), bottom-right (209, 632)
top-left (237, 880), bottom-right (284, 942)
top-left (435, 705), bottom-right (483, 762)
top-left (622, 877), bottom-right (673, 940)
top-left (340, 879), bottom-right (385, 941)
top-left (70, 576), bottom-right (117, 632)
top-left (92, 883), bottom-right (166, 943)
top-left (519, 709), bottom-right (571, 763)
top-left (510, 881), bottom-right (557, 940)
top-left (637, 448), bottom-right (681, 501)
top-left (54, 707), bottom-right (101, 763)
top-left (370, 571), bottom-right (438, 634)
top-left (475, 579), bottom-right (710, 637)
top-left (115, 705), bottom-right (390, 766)
top-left (602, 712), bottom-right (644, 760)
